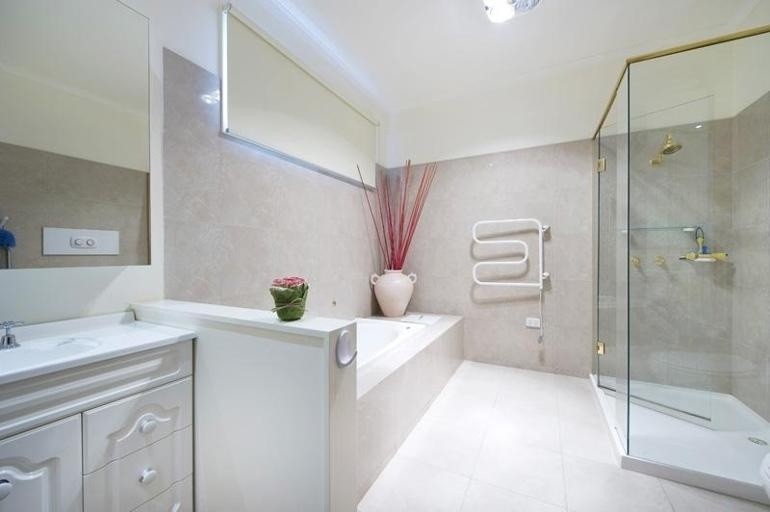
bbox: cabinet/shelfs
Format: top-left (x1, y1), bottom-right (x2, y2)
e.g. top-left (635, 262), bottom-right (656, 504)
top-left (1, 331), bottom-right (197, 512)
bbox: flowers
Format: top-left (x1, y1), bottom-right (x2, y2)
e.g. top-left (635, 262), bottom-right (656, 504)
top-left (271, 276), bottom-right (306, 290)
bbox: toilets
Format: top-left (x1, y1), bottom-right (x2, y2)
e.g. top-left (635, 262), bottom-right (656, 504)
top-left (758, 452), bottom-right (770, 499)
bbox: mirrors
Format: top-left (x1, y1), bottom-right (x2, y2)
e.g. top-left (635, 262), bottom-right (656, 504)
top-left (221, 0), bottom-right (380, 191)
top-left (0, 0), bottom-right (151, 270)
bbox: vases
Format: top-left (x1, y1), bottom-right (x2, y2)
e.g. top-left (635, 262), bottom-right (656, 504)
top-left (272, 285), bottom-right (306, 321)
top-left (369, 265), bottom-right (420, 319)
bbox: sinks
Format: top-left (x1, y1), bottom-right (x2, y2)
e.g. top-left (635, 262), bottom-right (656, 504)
top-left (0, 347), bottom-right (55, 374)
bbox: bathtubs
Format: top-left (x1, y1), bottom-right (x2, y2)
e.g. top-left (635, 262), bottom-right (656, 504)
top-left (355, 317), bottom-right (427, 372)
